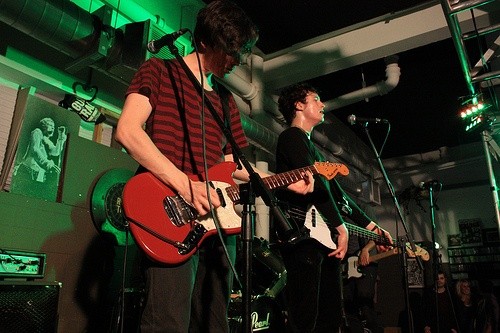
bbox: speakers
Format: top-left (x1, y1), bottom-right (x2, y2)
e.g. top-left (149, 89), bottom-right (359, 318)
top-left (0, 279), bottom-right (62, 333)
top-left (373, 243), bottom-right (437, 333)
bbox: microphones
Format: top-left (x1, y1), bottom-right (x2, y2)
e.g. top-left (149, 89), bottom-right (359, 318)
top-left (419, 180), bottom-right (441, 189)
top-left (348, 114), bottom-right (389, 124)
top-left (146, 28), bottom-right (188, 54)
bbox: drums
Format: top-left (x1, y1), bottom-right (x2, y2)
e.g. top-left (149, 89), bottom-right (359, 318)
top-left (229, 294), bottom-right (289, 333)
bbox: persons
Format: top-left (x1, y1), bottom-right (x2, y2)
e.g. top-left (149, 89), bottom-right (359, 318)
top-left (335, 218), bottom-right (385, 333)
top-left (269, 84), bottom-right (395, 333)
top-left (13, 116), bottom-right (67, 199)
top-left (423, 271), bottom-right (461, 333)
top-left (462, 262), bottom-right (493, 333)
top-left (113, 0), bottom-right (314, 333)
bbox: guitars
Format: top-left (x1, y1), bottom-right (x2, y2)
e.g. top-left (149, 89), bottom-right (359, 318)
top-left (121, 161), bottom-right (350, 266)
top-left (340, 247), bottom-right (403, 290)
top-left (274, 194), bottom-right (430, 262)
